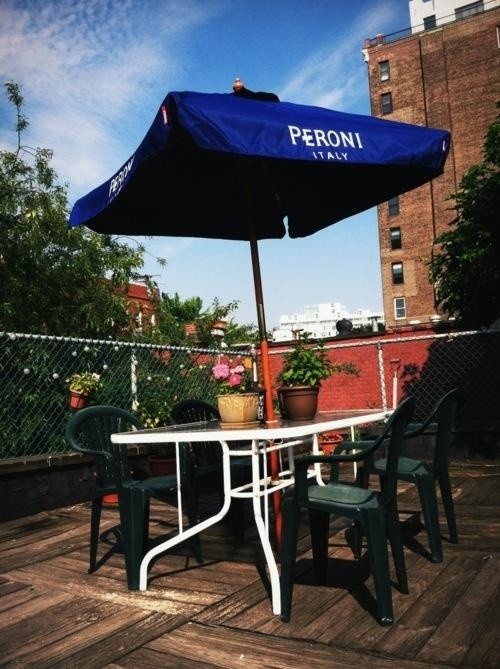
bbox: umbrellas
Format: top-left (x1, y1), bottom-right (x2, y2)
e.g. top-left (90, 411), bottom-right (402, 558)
top-left (61, 76), bottom-right (452, 559)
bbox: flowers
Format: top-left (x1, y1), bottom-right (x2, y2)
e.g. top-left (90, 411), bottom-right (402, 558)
top-left (69, 372), bottom-right (100, 396)
top-left (211, 354), bottom-right (254, 394)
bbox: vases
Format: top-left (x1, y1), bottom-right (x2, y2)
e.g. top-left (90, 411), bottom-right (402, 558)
top-left (215, 393), bottom-right (260, 429)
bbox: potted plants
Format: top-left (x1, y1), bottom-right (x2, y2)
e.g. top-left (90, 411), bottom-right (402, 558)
top-left (68, 393), bottom-right (88, 409)
top-left (149, 443), bottom-right (177, 476)
top-left (275, 326), bottom-right (329, 422)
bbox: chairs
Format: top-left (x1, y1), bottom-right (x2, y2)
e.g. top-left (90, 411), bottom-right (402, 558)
top-left (356, 388), bottom-right (464, 563)
top-left (64, 406), bottom-right (208, 572)
top-left (281, 397), bottom-right (417, 626)
top-left (169, 399), bottom-right (272, 550)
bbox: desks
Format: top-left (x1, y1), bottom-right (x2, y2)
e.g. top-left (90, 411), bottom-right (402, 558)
top-left (109, 409), bottom-right (395, 616)
